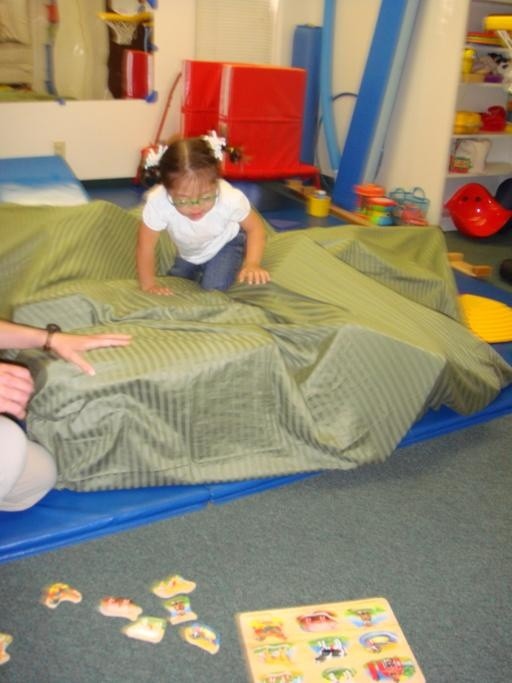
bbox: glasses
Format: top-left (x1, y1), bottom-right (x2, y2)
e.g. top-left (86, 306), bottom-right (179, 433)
top-left (167, 190), bottom-right (220, 207)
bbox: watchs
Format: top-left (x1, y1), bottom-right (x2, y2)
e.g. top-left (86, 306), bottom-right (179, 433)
top-left (44, 323), bottom-right (61, 352)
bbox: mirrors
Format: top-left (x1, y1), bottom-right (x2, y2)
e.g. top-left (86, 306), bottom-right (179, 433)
top-left (0, 0), bottom-right (155, 102)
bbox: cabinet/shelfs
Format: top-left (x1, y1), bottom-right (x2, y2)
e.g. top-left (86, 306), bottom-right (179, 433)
top-left (365, 0), bottom-right (512, 233)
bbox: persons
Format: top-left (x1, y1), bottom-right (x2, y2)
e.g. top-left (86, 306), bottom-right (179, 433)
top-left (136, 127), bottom-right (271, 298)
top-left (1, 319), bottom-right (134, 511)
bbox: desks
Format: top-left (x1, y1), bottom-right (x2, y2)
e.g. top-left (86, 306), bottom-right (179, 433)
top-left (0, 155), bottom-right (92, 205)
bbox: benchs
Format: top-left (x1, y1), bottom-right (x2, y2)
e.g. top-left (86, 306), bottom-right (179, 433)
top-left (216, 63), bottom-right (321, 188)
top-left (182, 59), bottom-right (267, 139)
